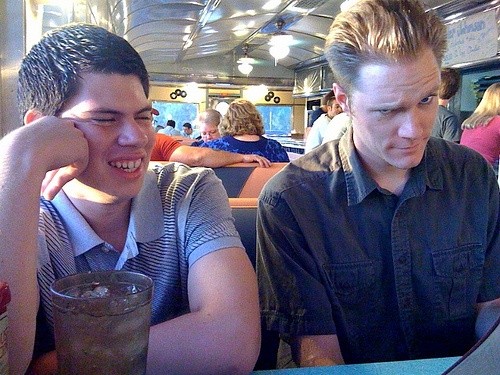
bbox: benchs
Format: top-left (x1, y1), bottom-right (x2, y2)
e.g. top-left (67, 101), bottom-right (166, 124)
top-left (211, 162), bottom-right (289, 269)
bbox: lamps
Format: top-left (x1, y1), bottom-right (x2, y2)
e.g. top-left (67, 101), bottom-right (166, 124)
top-left (236, 44), bottom-right (255, 75)
top-left (269, 19), bottom-right (294, 59)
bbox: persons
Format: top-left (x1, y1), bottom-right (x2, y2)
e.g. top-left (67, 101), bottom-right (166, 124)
top-left (458, 82), bottom-right (500, 167)
top-left (149, 98), bottom-right (290, 168)
top-left (304, 90), bottom-right (351, 156)
top-left (0, 21), bottom-right (261, 375)
top-left (256, 0), bottom-right (500, 375)
top-left (430, 67), bottom-right (460, 143)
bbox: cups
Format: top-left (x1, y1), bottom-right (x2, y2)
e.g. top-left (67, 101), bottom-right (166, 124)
top-left (0, 279), bottom-right (12, 375)
top-left (49, 270), bottom-right (154, 375)
